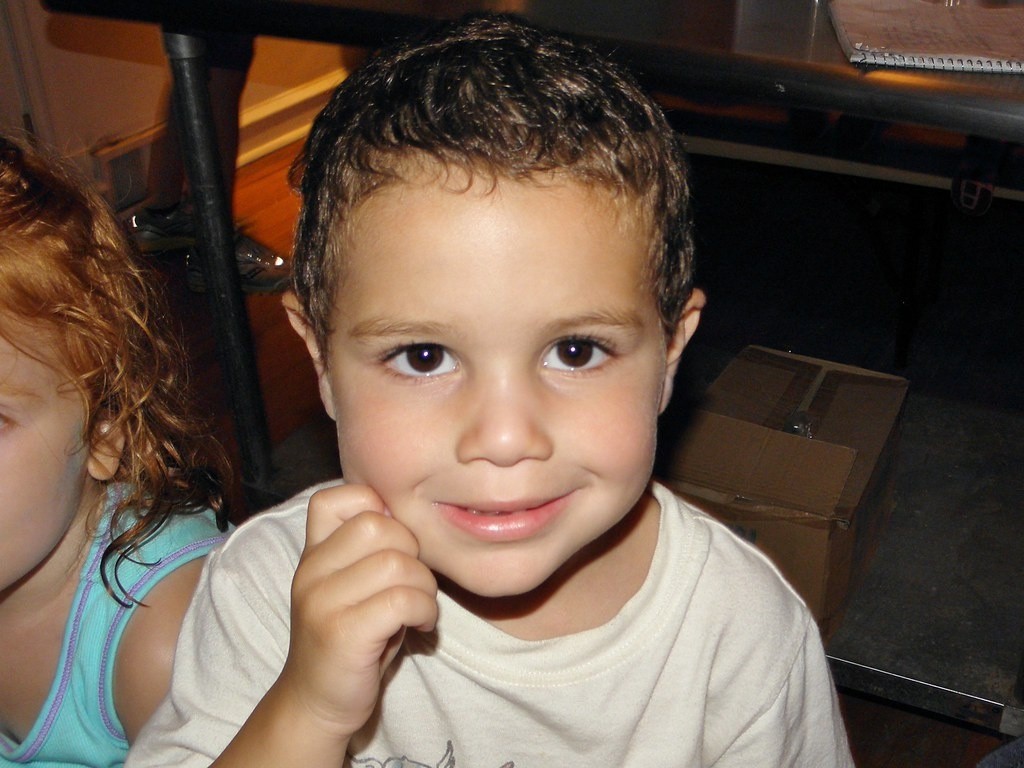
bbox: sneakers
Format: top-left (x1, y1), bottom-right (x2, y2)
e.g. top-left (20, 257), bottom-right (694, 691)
top-left (186, 227), bottom-right (294, 294)
top-left (128, 201), bottom-right (196, 257)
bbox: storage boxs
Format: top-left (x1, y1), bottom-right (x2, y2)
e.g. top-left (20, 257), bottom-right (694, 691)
top-left (670, 343), bottom-right (914, 648)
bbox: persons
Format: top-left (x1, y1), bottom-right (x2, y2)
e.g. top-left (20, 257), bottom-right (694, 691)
top-left (120, 14), bottom-right (855, 768)
top-left (0, 124), bottom-right (240, 767)
